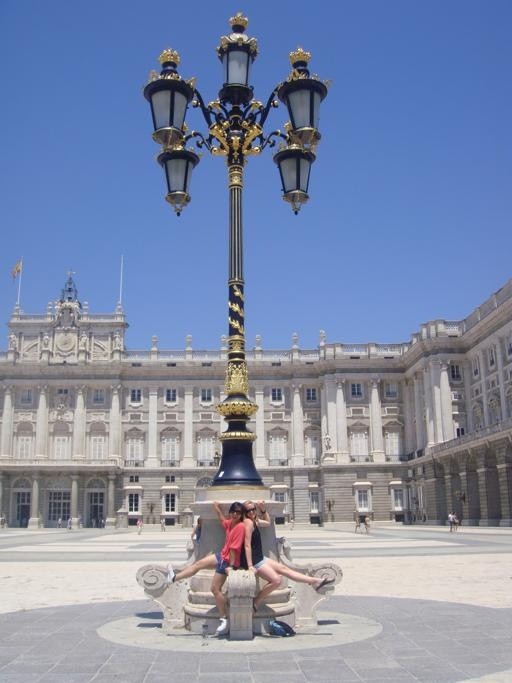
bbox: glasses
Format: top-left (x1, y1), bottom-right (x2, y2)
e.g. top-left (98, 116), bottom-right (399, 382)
top-left (230, 509), bottom-right (242, 515)
top-left (247, 508), bottom-right (256, 512)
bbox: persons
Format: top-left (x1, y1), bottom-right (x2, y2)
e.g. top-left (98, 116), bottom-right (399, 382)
top-left (92, 517), bottom-right (95, 528)
top-left (447, 511), bottom-right (453, 532)
top-left (160, 518), bottom-right (166, 532)
top-left (452, 511), bottom-right (459, 532)
top-left (355, 513), bottom-right (362, 534)
top-left (67, 517), bottom-right (71, 529)
top-left (364, 514), bottom-right (372, 534)
top-left (191, 516), bottom-right (201, 555)
top-left (289, 516), bottom-right (295, 532)
top-left (58, 518), bottom-right (62, 527)
top-left (137, 518), bottom-right (144, 535)
top-left (244, 498), bottom-right (337, 611)
top-left (165, 501), bottom-right (246, 635)
top-left (102, 517), bottom-right (105, 527)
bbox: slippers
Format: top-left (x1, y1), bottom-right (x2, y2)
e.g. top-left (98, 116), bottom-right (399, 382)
top-left (253, 597), bottom-right (259, 612)
top-left (315, 576), bottom-right (336, 591)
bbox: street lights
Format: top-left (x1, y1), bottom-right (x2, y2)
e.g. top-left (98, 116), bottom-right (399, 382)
top-left (138, 14), bottom-right (335, 487)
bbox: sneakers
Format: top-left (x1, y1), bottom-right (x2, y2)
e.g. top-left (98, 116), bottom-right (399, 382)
top-left (166, 563), bottom-right (176, 585)
top-left (216, 616), bottom-right (229, 633)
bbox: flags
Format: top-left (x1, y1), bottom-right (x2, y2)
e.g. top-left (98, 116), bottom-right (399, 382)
top-left (9, 256), bottom-right (23, 279)
